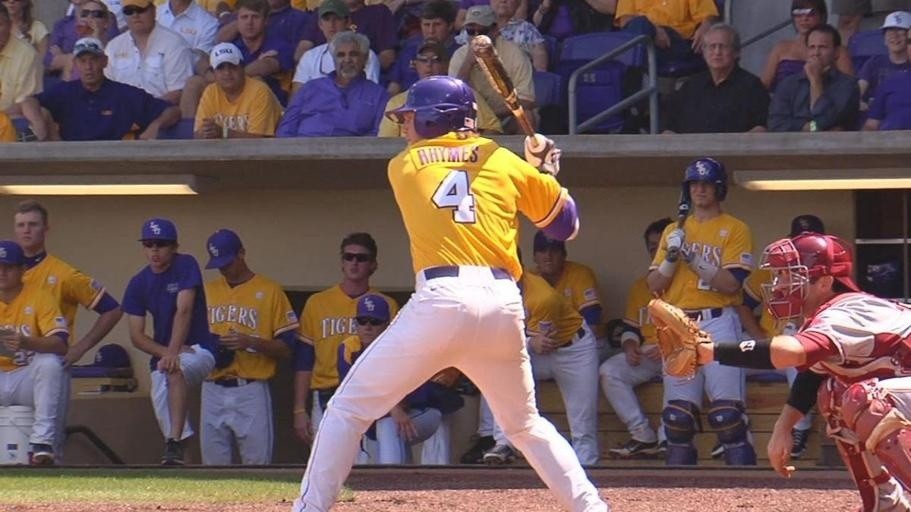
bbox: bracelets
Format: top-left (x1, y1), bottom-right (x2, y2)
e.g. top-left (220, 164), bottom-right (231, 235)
top-left (710, 337), bottom-right (777, 371)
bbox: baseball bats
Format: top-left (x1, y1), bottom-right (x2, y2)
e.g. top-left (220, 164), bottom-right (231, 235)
top-left (665, 190), bottom-right (690, 257)
top-left (472, 35), bottom-right (557, 164)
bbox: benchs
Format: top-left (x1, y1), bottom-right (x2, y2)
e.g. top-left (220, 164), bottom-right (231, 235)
top-left (532, 378), bottom-right (825, 469)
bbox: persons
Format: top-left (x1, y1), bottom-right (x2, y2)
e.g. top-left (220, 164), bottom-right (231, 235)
top-left (300, 231), bottom-right (466, 467)
top-left (466, 221), bottom-right (602, 471)
top-left (0, 2), bottom-right (911, 142)
top-left (597, 157), bottom-right (759, 467)
top-left (0, 204), bottom-right (122, 472)
top-left (646, 228), bottom-right (911, 510)
top-left (746, 216), bottom-right (828, 460)
top-left (123, 217), bottom-right (301, 466)
top-left (290, 72), bottom-right (615, 512)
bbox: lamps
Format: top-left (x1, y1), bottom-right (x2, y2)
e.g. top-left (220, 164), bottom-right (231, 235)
top-left (0, 175), bottom-right (200, 195)
top-left (732, 167), bottom-right (911, 191)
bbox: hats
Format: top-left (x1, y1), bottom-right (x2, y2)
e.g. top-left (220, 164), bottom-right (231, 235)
top-left (1, 238), bottom-right (26, 265)
top-left (880, 7), bottom-right (911, 32)
top-left (210, 43), bottom-right (244, 68)
top-left (417, 36), bottom-right (452, 59)
top-left (86, 343), bottom-right (129, 370)
top-left (532, 229), bottom-right (569, 251)
top-left (72, 36), bottom-right (106, 57)
top-left (464, 6), bottom-right (497, 29)
top-left (138, 216), bottom-right (178, 244)
top-left (353, 296), bottom-right (387, 321)
top-left (204, 230), bottom-right (240, 268)
top-left (320, 1), bottom-right (349, 20)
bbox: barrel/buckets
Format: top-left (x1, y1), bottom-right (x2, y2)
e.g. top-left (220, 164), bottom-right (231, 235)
top-left (0, 404), bottom-right (35, 466)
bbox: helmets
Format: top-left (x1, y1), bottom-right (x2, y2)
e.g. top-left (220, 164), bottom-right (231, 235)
top-left (684, 158), bottom-right (728, 183)
top-left (385, 72), bottom-right (479, 136)
top-left (760, 232), bottom-right (853, 316)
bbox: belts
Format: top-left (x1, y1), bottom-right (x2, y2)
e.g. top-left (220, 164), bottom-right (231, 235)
top-left (686, 306), bottom-right (725, 321)
top-left (417, 263), bottom-right (514, 282)
top-left (210, 374), bottom-right (255, 387)
top-left (560, 327), bottom-right (585, 345)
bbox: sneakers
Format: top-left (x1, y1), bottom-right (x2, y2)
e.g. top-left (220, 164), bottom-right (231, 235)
top-left (477, 435), bottom-right (515, 462)
top-left (789, 423), bottom-right (811, 459)
top-left (159, 438), bottom-right (183, 464)
top-left (29, 443), bottom-right (57, 466)
top-left (608, 430), bottom-right (727, 458)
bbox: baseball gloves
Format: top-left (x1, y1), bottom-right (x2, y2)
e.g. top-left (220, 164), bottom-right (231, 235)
top-left (649, 298), bottom-right (704, 378)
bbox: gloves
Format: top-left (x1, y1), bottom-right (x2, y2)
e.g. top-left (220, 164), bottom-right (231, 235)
top-left (663, 228), bottom-right (697, 260)
top-left (521, 134), bottom-right (562, 178)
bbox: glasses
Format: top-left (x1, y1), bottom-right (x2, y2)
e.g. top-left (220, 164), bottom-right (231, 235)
top-left (342, 249), bottom-right (372, 264)
top-left (467, 27), bottom-right (493, 35)
top-left (80, 4), bottom-right (150, 19)
top-left (338, 48), bottom-right (363, 59)
top-left (141, 237), bottom-right (169, 248)
top-left (418, 55), bottom-right (446, 62)
top-left (355, 316), bottom-right (381, 325)
